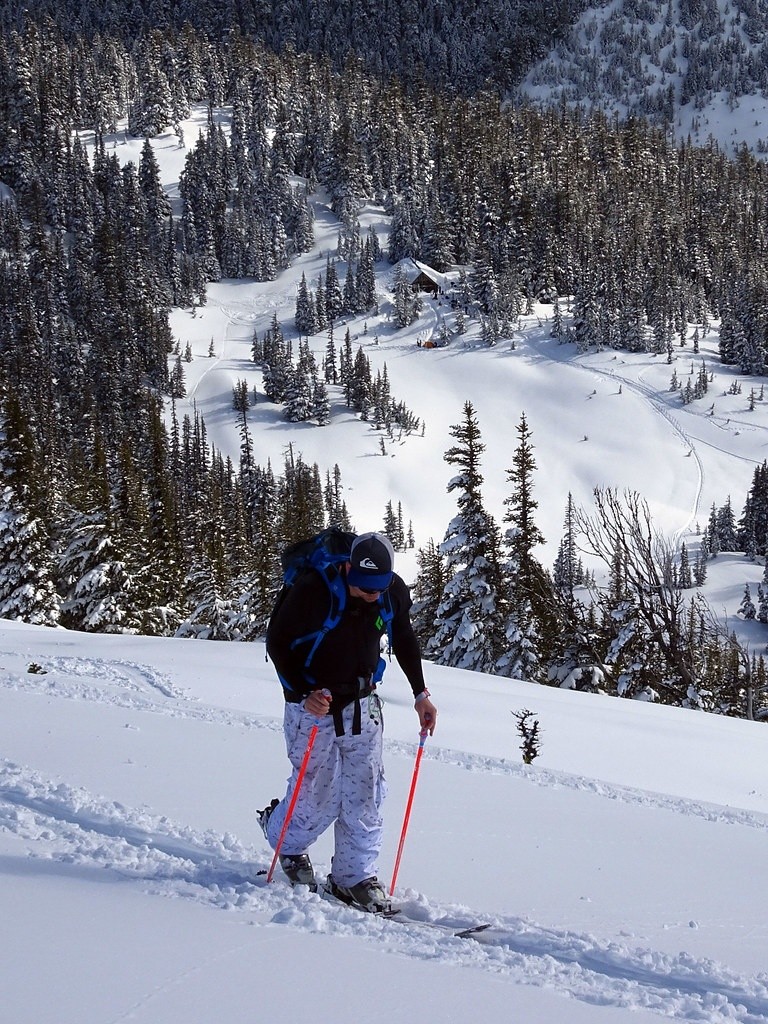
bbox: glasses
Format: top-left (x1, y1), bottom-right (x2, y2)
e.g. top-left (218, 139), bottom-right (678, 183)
top-left (359, 573), bottom-right (396, 594)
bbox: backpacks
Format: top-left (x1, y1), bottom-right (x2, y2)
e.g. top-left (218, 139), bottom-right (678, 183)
top-left (280, 523), bottom-right (393, 666)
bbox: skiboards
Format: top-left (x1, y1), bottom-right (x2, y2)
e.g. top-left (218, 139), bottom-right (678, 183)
top-left (324, 890), bottom-right (492, 942)
top-left (260, 804), bottom-right (320, 899)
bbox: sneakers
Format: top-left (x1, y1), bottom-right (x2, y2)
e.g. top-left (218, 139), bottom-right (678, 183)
top-left (326, 874), bottom-right (393, 913)
top-left (264, 807), bottom-right (315, 884)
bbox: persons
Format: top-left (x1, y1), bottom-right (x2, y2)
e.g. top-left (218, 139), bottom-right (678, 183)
top-left (265, 532), bottom-right (437, 918)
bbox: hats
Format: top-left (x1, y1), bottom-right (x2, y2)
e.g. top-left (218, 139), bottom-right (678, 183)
top-left (346, 532), bottom-right (395, 590)
